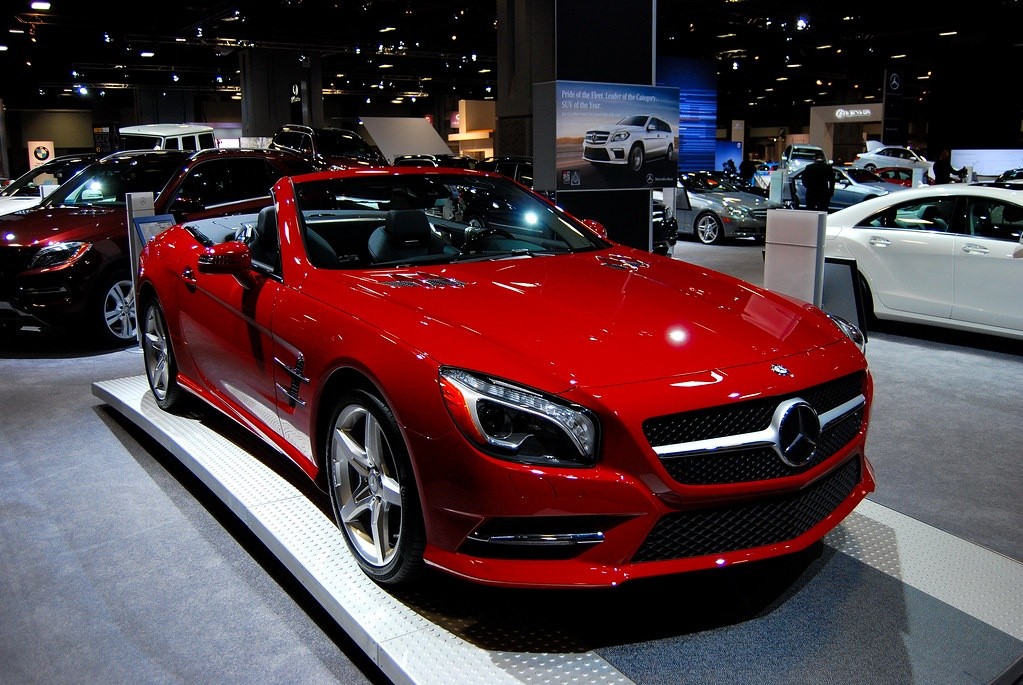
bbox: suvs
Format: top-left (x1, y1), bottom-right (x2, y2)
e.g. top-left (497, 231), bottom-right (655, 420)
top-left (0, 124), bottom-right (534, 264)
top-left (0, 147), bottom-right (395, 348)
top-left (582, 114), bottom-right (676, 173)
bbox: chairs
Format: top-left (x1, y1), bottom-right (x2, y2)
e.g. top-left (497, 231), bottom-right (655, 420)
top-left (918, 205), bottom-right (949, 232)
top-left (252, 203), bottom-right (342, 276)
top-left (879, 208), bottom-right (904, 229)
top-left (366, 211), bottom-right (461, 272)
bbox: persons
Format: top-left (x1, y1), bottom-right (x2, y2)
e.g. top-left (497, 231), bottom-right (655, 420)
top-left (932, 150), bottom-right (959, 185)
top-left (739, 156), bottom-right (757, 184)
top-left (802, 154), bottom-right (835, 210)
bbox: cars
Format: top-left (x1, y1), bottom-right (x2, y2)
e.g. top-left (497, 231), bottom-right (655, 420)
top-left (650, 169), bottom-right (790, 245)
top-left (716, 144), bottom-right (1023, 236)
top-left (824, 180), bottom-right (1023, 340)
top-left (651, 198), bottom-right (679, 258)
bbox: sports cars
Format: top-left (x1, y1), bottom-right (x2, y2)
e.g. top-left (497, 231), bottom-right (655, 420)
top-left (135, 166), bottom-right (877, 592)
